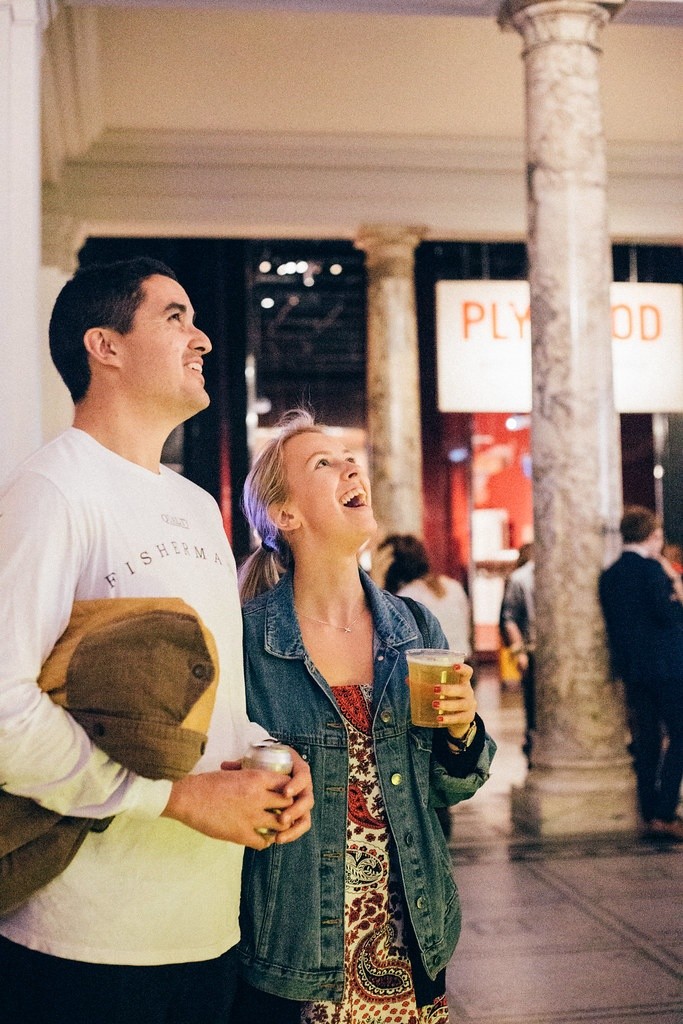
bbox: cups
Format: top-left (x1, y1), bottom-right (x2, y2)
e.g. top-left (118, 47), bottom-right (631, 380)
top-left (405, 648), bottom-right (466, 727)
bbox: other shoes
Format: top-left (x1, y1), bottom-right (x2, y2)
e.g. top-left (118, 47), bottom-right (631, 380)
top-left (640, 815), bottom-right (683, 847)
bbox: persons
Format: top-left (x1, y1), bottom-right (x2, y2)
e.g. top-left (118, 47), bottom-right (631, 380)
top-left (499, 542), bottom-right (535, 772)
top-left (241, 409), bottom-right (498, 1024)
top-left (596, 505), bottom-right (683, 845)
top-left (0, 256), bottom-right (314, 1024)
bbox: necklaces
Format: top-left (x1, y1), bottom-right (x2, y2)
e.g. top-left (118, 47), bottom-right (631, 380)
top-left (294, 604), bottom-right (368, 633)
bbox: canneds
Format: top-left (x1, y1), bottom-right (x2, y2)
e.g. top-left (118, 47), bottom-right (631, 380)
top-left (239, 741), bottom-right (292, 846)
